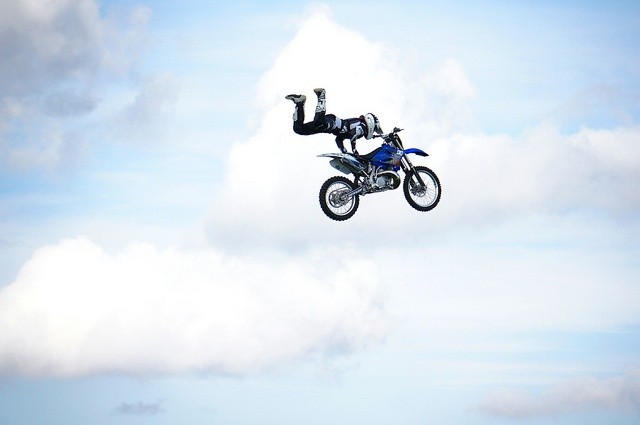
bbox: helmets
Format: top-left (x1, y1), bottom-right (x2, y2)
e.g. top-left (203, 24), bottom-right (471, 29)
top-left (364, 113), bottom-right (384, 140)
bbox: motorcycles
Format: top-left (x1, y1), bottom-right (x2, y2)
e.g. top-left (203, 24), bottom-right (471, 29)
top-left (316, 127), bottom-right (441, 221)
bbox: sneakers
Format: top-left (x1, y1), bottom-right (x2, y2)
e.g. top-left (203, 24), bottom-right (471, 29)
top-left (314, 88), bottom-right (325, 98)
top-left (285, 94), bottom-right (306, 104)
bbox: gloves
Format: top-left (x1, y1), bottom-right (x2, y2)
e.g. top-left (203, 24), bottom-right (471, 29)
top-left (352, 149), bottom-right (359, 157)
top-left (342, 148), bottom-right (347, 153)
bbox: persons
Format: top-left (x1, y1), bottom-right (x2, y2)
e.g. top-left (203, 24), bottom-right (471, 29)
top-left (285, 87), bottom-right (384, 156)
